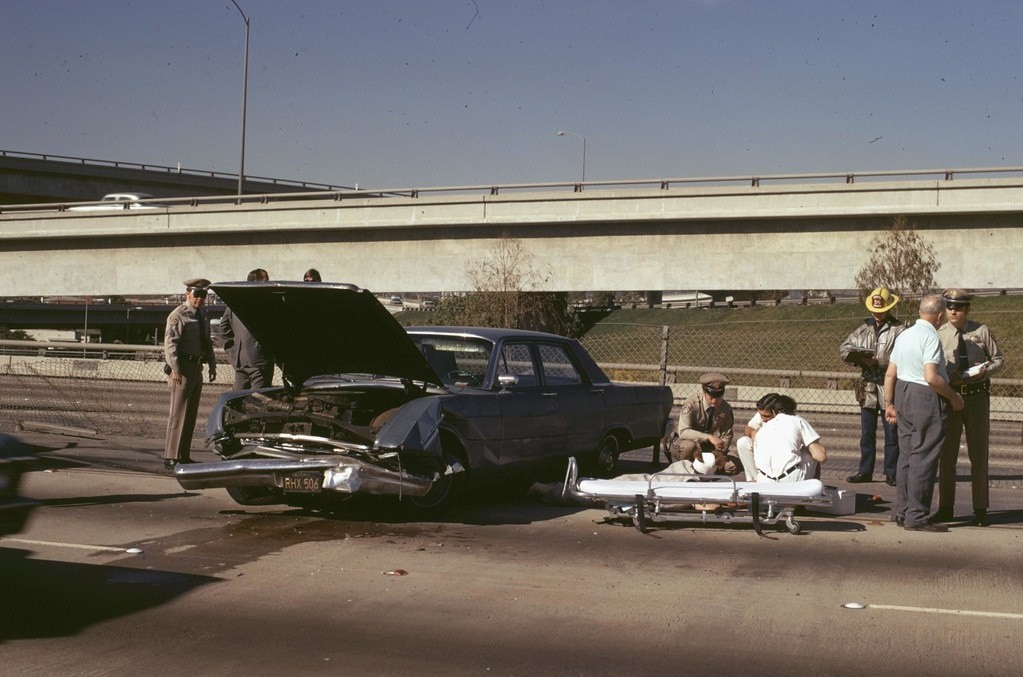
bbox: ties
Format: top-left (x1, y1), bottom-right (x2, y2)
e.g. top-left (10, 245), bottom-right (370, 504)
top-left (956, 329), bottom-right (970, 372)
top-left (705, 407), bottom-right (714, 449)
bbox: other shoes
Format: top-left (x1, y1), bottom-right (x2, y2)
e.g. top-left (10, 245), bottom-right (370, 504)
top-left (905, 519), bottom-right (949, 532)
top-left (179, 458), bottom-right (203, 464)
top-left (974, 509), bottom-right (984, 526)
top-left (896, 515), bottom-right (905, 526)
top-left (927, 512), bottom-right (953, 522)
top-left (165, 459), bottom-right (182, 470)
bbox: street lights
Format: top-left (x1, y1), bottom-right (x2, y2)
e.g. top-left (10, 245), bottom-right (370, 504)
top-left (556, 130), bottom-right (586, 190)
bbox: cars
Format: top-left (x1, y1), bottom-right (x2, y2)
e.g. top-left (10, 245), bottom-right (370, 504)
top-left (171, 276), bottom-right (679, 516)
top-left (65, 193), bottom-right (166, 215)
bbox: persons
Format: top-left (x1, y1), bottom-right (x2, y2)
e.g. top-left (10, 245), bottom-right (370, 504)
top-left (753, 396), bottom-right (827, 483)
top-left (664, 372), bottom-right (741, 476)
top-left (220, 269), bottom-right (274, 391)
top-left (883, 294), bottom-right (964, 531)
top-left (303, 268), bottom-right (321, 284)
top-left (929, 288), bottom-right (1005, 524)
top-left (840, 286), bottom-right (908, 484)
top-left (736, 392), bottom-right (780, 482)
top-left (528, 449), bottom-right (717, 511)
top-left (163, 278), bottom-right (217, 471)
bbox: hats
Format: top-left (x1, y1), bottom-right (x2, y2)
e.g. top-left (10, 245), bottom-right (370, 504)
top-left (942, 288), bottom-right (976, 310)
top-left (699, 373), bottom-right (730, 397)
top-left (182, 278), bottom-right (211, 290)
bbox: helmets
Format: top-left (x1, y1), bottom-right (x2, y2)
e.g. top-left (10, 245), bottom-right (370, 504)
top-left (866, 287), bottom-right (899, 313)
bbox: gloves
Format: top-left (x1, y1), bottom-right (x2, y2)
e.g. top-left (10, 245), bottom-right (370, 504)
top-left (851, 352), bottom-right (879, 369)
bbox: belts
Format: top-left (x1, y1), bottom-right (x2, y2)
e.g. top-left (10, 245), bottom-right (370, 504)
top-left (951, 381), bottom-right (988, 396)
top-left (759, 464), bottom-right (800, 481)
top-left (177, 348), bottom-right (206, 365)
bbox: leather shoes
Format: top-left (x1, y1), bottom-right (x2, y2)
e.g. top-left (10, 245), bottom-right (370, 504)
top-left (886, 475), bottom-right (896, 486)
top-left (846, 473), bottom-right (872, 482)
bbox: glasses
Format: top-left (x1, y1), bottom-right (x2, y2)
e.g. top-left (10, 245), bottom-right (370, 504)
top-left (193, 290), bottom-right (207, 298)
top-left (304, 280), bottom-right (313, 282)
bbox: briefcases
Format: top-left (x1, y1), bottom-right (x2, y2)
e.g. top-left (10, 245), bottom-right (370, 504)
top-left (804, 485), bottom-right (856, 515)
